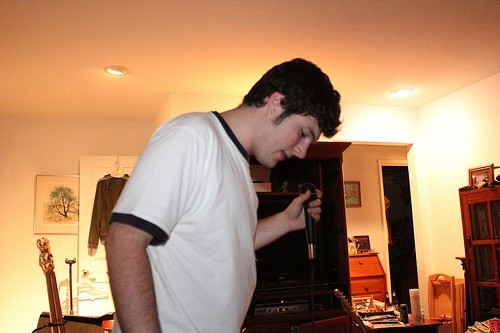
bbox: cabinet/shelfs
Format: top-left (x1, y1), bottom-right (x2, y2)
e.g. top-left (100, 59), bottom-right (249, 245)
top-left (347, 253), bottom-right (388, 306)
top-left (240, 141), bottom-right (356, 332)
top-left (458, 184), bottom-right (500, 324)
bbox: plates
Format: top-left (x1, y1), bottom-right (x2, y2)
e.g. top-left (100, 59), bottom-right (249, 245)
top-left (363, 314), bottom-right (399, 324)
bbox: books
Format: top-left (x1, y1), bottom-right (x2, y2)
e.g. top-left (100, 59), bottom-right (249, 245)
top-left (357, 310), bottom-right (406, 330)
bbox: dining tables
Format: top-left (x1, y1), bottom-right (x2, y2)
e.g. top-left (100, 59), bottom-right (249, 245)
top-left (352, 297), bottom-right (443, 332)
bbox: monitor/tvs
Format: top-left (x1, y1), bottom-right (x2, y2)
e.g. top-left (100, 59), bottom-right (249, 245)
top-left (255, 192), bottom-right (321, 289)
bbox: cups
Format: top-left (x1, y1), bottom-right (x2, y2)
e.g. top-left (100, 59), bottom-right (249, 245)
top-left (409, 308), bottom-right (425, 325)
top-left (400, 304), bottom-right (408, 323)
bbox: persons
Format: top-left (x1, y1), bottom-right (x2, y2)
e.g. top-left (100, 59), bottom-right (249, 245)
top-left (104, 58), bottom-right (343, 333)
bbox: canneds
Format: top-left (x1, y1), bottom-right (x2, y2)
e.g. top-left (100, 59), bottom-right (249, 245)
top-left (489, 318), bottom-right (500, 333)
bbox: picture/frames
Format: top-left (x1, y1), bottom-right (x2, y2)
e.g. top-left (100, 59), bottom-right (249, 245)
top-left (33, 173), bottom-right (80, 235)
top-left (343, 180), bottom-right (362, 208)
top-left (354, 235), bottom-right (370, 252)
top-left (468, 164), bottom-right (494, 186)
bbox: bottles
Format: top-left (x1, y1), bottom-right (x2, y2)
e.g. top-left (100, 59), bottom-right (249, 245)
top-left (391, 290), bottom-right (398, 309)
top-left (385, 292), bottom-right (391, 311)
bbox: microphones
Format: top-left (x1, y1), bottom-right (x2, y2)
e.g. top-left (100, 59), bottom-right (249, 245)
top-left (298, 183), bottom-right (317, 260)
